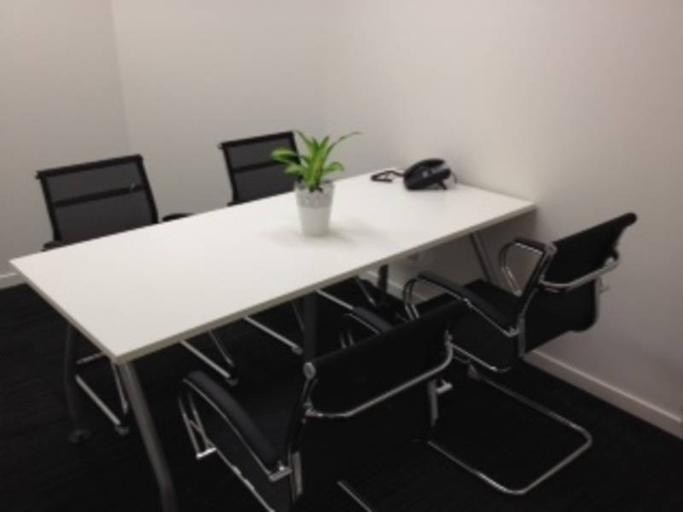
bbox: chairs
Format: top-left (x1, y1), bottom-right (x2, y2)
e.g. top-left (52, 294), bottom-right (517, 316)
top-left (186, 278), bottom-right (466, 511)
top-left (400, 211), bottom-right (639, 494)
top-left (218, 131), bottom-right (308, 208)
top-left (34, 149), bottom-right (205, 449)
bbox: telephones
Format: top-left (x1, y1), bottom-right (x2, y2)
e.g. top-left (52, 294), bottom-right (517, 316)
top-left (403, 159), bottom-right (451, 190)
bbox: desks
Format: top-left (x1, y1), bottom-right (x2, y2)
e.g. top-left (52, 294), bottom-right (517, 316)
top-left (9, 169), bottom-right (537, 508)
